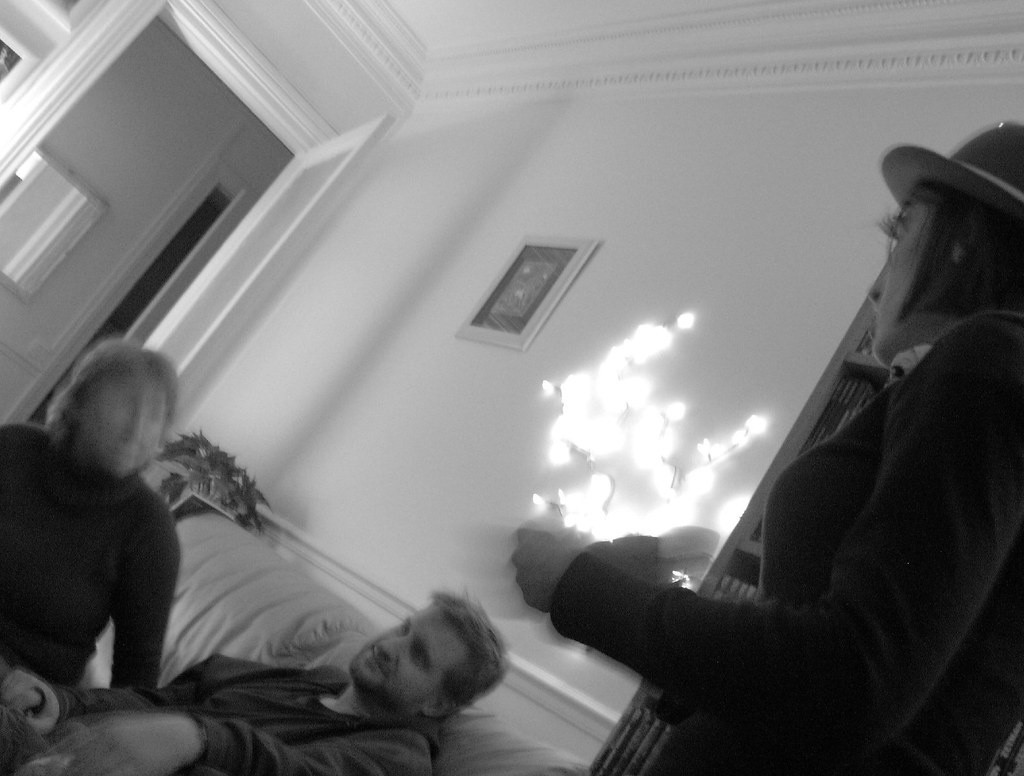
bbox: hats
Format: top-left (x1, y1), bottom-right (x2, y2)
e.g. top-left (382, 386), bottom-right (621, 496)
top-left (880, 123), bottom-right (1024, 223)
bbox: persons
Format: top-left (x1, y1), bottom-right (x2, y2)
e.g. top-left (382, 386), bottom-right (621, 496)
top-left (0, 590), bottom-right (510, 776)
top-left (0, 339), bottom-right (182, 689)
top-left (512, 121), bottom-right (1024, 775)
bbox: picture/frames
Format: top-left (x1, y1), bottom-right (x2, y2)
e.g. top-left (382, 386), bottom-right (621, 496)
top-left (455, 233), bottom-right (601, 352)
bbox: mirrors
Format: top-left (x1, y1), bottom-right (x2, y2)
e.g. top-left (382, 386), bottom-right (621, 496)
top-left (0, 147), bottom-right (109, 304)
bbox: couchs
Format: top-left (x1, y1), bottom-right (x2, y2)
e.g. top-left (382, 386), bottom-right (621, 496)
top-left (84, 512), bottom-right (590, 776)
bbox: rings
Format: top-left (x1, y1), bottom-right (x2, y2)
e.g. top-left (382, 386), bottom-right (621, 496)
top-left (29, 687), bottom-right (46, 713)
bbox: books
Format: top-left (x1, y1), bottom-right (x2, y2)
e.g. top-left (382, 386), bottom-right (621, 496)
top-left (712, 574), bottom-right (757, 602)
top-left (800, 374), bottom-right (876, 455)
top-left (983, 719), bottom-right (1024, 776)
top-left (594, 707), bottom-right (674, 776)
